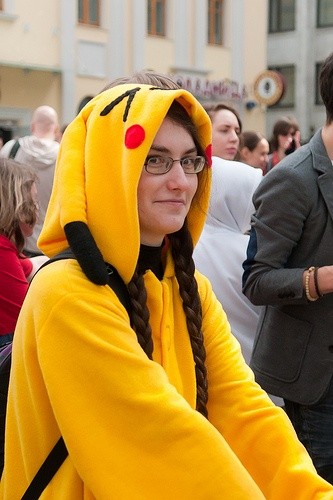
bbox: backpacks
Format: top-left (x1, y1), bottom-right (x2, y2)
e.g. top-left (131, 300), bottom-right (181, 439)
top-left (0, 247), bottom-right (131, 500)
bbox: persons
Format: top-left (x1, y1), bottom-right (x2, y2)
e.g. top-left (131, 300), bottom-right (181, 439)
top-left (0, 53), bottom-right (333, 500)
top-left (0, 83), bottom-right (333, 500)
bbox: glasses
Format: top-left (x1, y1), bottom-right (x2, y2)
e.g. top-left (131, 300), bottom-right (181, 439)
top-left (144, 155), bottom-right (208, 175)
top-left (281, 132), bottom-right (295, 136)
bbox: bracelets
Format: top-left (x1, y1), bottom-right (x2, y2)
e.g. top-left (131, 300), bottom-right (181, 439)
top-left (304, 266), bottom-right (318, 301)
top-left (314, 266), bottom-right (326, 298)
